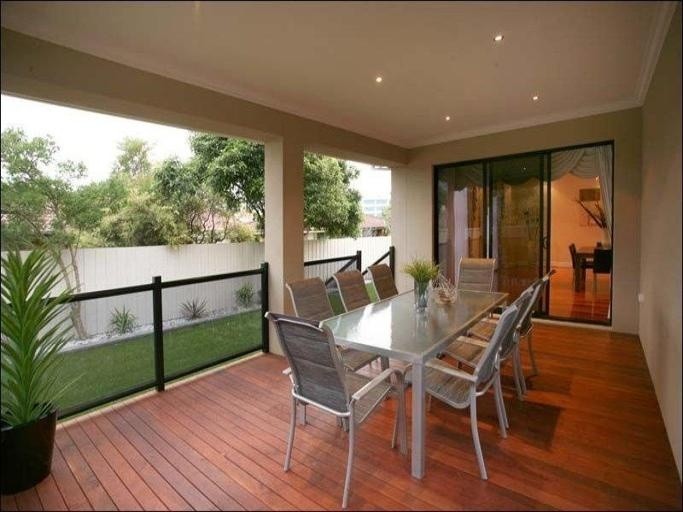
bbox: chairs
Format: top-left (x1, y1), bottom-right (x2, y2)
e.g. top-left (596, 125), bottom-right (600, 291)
top-left (390, 306), bottom-right (509, 482)
top-left (330, 267), bottom-right (374, 314)
top-left (569, 243), bottom-right (613, 296)
top-left (283, 275), bottom-right (388, 432)
top-left (439, 256), bottom-right (502, 359)
top-left (461, 277), bottom-right (544, 406)
top-left (367, 261), bottom-right (405, 302)
top-left (426, 292), bottom-right (529, 438)
top-left (263, 310), bottom-right (407, 508)
top-left (482, 267), bottom-right (556, 379)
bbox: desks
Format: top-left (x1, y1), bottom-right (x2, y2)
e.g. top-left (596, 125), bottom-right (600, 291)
top-left (312, 284), bottom-right (513, 480)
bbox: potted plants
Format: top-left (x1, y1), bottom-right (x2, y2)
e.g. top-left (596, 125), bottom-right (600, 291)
top-left (577, 199), bottom-right (611, 248)
top-left (0, 236), bottom-right (86, 497)
top-left (400, 256), bottom-right (446, 313)
